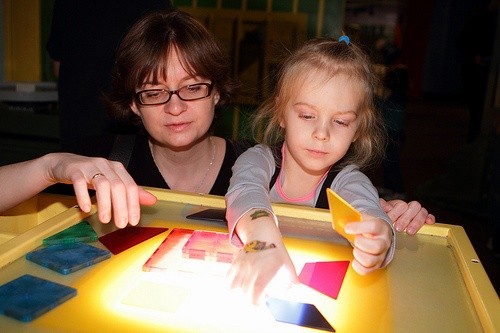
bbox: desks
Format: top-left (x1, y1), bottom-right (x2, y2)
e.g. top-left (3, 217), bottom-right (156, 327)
top-left (0, 186), bottom-right (500, 333)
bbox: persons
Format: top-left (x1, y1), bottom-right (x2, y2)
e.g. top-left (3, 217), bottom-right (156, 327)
top-left (377, 42), bottom-right (411, 200)
top-left (0, 11), bottom-right (435, 237)
top-left (224, 37), bottom-right (395, 302)
top-left (46, 0), bottom-right (173, 196)
top-left (457, 49), bottom-right (491, 141)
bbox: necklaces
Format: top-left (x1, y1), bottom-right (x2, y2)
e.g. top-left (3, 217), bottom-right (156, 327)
top-left (150, 137), bottom-right (216, 192)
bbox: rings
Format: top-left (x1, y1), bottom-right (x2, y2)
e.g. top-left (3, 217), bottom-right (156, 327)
top-left (90, 173), bottom-right (105, 183)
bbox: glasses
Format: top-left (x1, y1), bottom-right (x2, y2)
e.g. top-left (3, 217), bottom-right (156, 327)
top-left (132, 80), bottom-right (214, 106)
